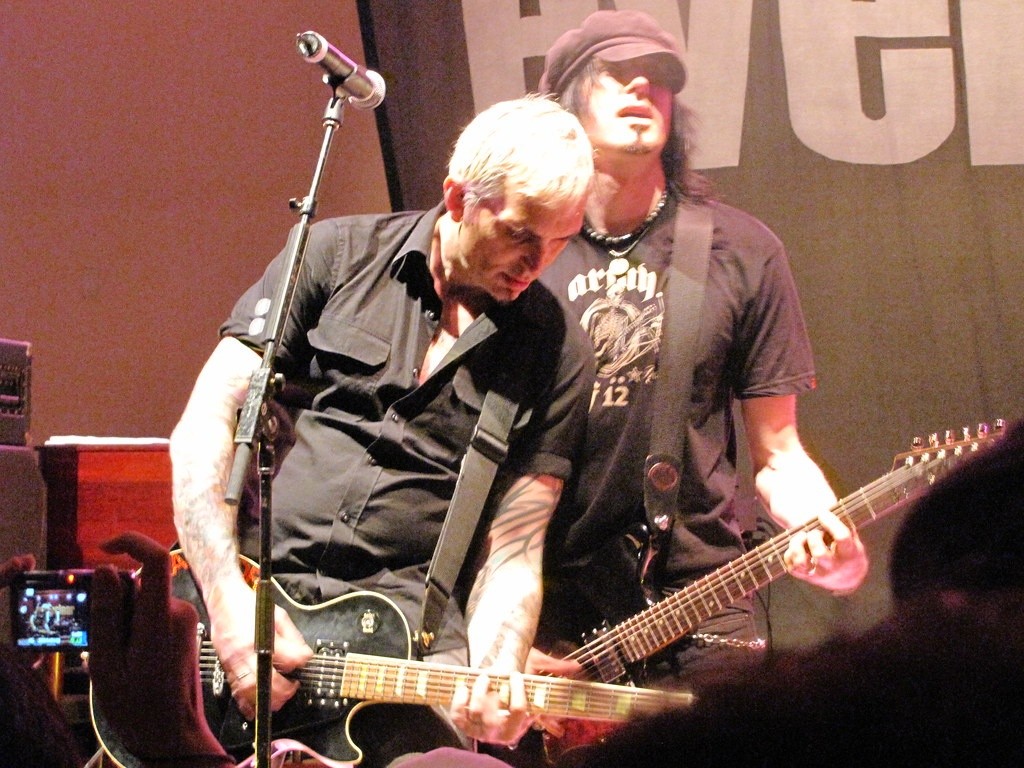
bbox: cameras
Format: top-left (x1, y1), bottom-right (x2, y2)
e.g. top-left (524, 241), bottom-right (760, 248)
top-left (8, 567), bottom-right (135, 653)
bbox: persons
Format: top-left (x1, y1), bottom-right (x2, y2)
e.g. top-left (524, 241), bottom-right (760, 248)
top-left (0, 531), bottom-right (239, 768)
top-left (169, 94), bottom-right (597, 746)
top-left (521, 12), bottom-right (869, 733)
top-left (395, 416), bottom-right (1024, 768)
top-left (25, 593), bottom-right (85, 644)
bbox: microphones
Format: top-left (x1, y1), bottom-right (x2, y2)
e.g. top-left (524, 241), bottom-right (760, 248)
top-left (295, 30), bottom-right (387, 111)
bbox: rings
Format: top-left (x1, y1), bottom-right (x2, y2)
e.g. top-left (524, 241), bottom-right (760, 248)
top-left (805, 560), bottom-right (817, 575)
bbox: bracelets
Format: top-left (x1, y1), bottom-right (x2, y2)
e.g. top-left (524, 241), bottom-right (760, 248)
top-left (142, 755), bottom-right (237, 768)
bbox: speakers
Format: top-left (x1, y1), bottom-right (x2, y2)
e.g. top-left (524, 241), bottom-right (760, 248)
top-left (0, 439), bottom-right (52, 651)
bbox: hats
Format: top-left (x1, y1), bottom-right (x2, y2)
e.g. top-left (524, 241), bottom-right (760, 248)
top-left (538, 10), bottom-right (688, 102)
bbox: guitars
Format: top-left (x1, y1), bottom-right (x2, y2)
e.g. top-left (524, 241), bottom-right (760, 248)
top-left (86, 547), bottom-right (698, 768)
top-left (521, 417), bottom-right (1009, 768)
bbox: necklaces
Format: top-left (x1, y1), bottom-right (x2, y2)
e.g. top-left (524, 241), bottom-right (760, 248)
top-left (583, 181), bottom-right (669, 244)
